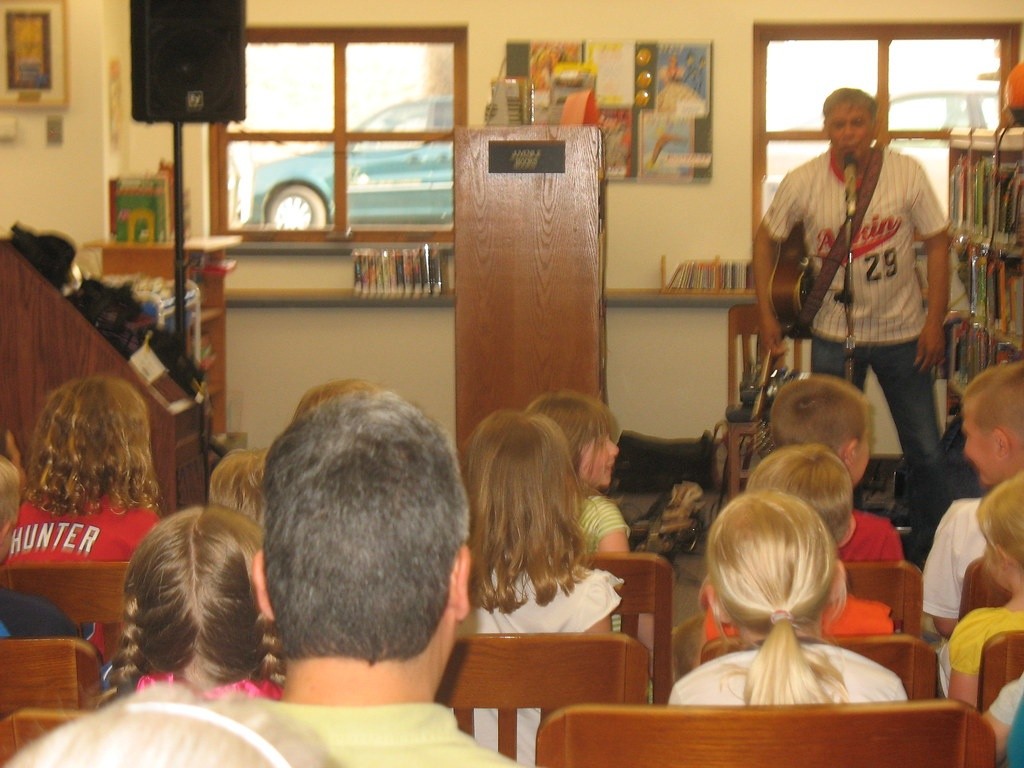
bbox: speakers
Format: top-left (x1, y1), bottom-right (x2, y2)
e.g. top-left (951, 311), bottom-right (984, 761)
top-left (128, 0), bottom-right (247, 124)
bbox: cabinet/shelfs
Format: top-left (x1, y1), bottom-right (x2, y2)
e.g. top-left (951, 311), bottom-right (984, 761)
top-left (86, 235), bottom-right (243, 467)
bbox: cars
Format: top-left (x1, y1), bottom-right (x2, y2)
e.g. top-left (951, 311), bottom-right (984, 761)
top-left (228, 97), bottom-right (454, 230)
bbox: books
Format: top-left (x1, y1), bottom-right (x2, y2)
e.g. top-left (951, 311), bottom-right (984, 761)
top-left (116, 178), bottom-right (171, 245)
top-left (352, 242), bottom-right (442, 300)
top-left (951, 151), bottom-right (1024, 393)
top-left (666, 261), bottom-right (749, 292)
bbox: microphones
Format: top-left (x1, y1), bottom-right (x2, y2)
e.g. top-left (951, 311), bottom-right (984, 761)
top-left (843, 148), bottom-right (856, 164)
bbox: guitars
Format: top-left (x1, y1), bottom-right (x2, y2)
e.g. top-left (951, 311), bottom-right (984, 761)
top-left (736, 220), bottom-right (816, 491)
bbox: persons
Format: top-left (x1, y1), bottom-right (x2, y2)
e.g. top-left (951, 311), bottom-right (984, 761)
top-left (203, 376), bottom-right (533, 767)
top-left (15, 375), bottom-right (165, 665)
top-left (915, 362), bottom-right (1024, 703)
top-left (769, 377), bottom-right (906, 564)
top-left (454, 406), bottom-right (616, 768)
top-left (695, 442), bottom-right (895, 671)
top-left (942, 471), bottom-right (1024, 768)
top-left (751, 87), bottom-right (953, 552)
top-left (1, 425), bottom-right (81, 642)
top-left (527, 389), bottom-right (632, 631)
top-left (94, 505), bottom-right (286, 700)
top-left (666, 489), bottom-right (908, 708)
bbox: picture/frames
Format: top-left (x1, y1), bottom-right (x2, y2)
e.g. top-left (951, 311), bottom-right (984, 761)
top-left (0, 0), bottom-right (71, 111)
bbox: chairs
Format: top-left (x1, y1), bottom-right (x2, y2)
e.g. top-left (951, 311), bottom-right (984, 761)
top-left (723, 305), bottom-right (802, 497)
top-left (0, 707), bottom-right (91, 768)
top-left (0, 561), bottom-right (132, 662)
top-left (434, 632), bottom-right (651, 762)
top-left (575, 553), bottom-right (673, 705)
top-left (535, 697), bottom-right (996, 768)
top-left (702, 633), bottom-right (938, 700)
top-left (0, 635), bottom-right (104, 720)
top-left (958, 557), bottom-right (1012, 623)
top-left (843, 561), bottom-right (923, 638)
top-left (981, 629), bottom-right (1024, 714)
